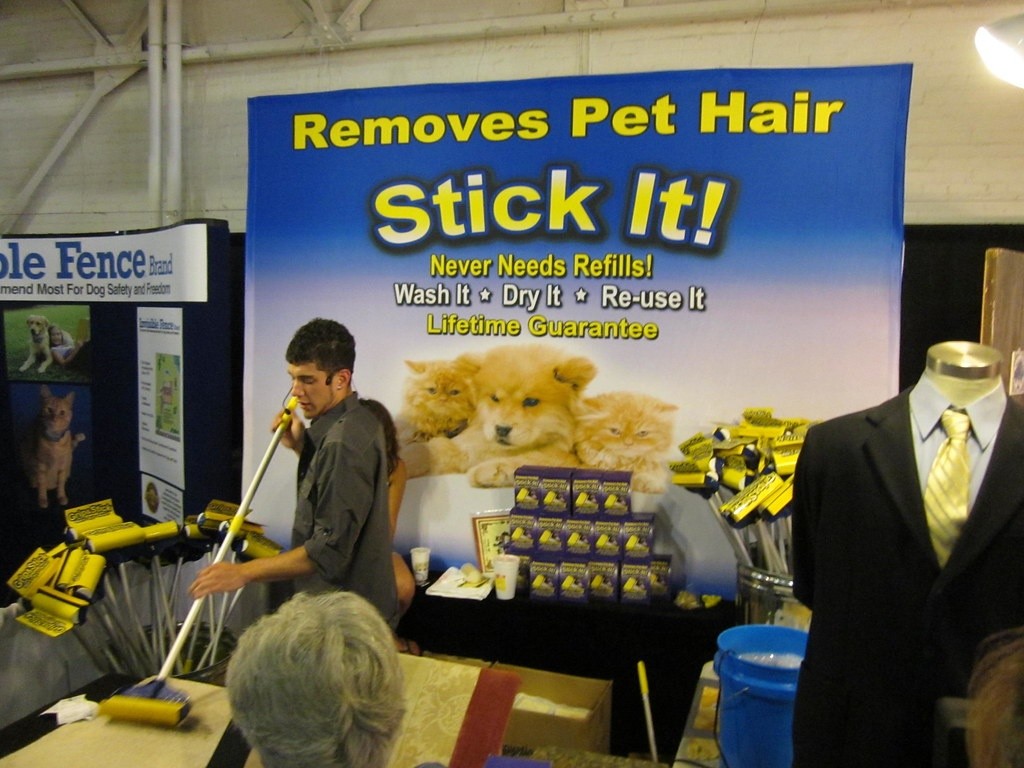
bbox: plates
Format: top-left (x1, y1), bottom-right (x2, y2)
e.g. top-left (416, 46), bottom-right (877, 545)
top-left (425, 566), bottom-right (495, 601)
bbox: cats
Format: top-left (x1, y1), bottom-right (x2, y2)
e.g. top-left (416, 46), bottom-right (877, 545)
top-left (575, 392), bottom-right (679, 494)
top-left (26, 384), bottom-right (86, 509)
top-left (394, 353), bottom-right (479, 448)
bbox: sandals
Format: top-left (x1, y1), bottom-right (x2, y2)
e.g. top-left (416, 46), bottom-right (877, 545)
top-left (398, 637), bottom-right (422, 658)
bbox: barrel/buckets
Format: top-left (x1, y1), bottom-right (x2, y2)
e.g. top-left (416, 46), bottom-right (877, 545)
top-left (711, 624), bottom-right (809, 768)
top-left (94, 620), bottom-right (238, 685)
top-left (735, 539), bottom-right (811, 631)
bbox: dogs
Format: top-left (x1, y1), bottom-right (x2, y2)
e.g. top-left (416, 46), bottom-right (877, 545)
top-left (18, 315), bottom-right (75, 374)
top-left (389, 343), bottom-right (599, 489)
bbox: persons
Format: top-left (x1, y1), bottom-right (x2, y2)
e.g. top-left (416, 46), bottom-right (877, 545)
top-left (187, 319), bottom-right (397, 624)
top-left (357, 398), bottom-right (423, 658)
top-left (225, 592), bottom-right (402, 767)
top-left (792, 341), bottom-right (1024, 768)
top-left (48, 325), bottom-right (92, 366)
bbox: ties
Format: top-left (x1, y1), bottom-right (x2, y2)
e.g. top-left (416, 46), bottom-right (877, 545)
top-left (923, 410), bottom-right (975, 566)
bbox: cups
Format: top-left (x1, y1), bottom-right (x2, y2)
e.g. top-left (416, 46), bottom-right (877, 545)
top-left (491, 553), bottom-right (520, 600)
top-left (409, 548), bottom-right (432, 585)
